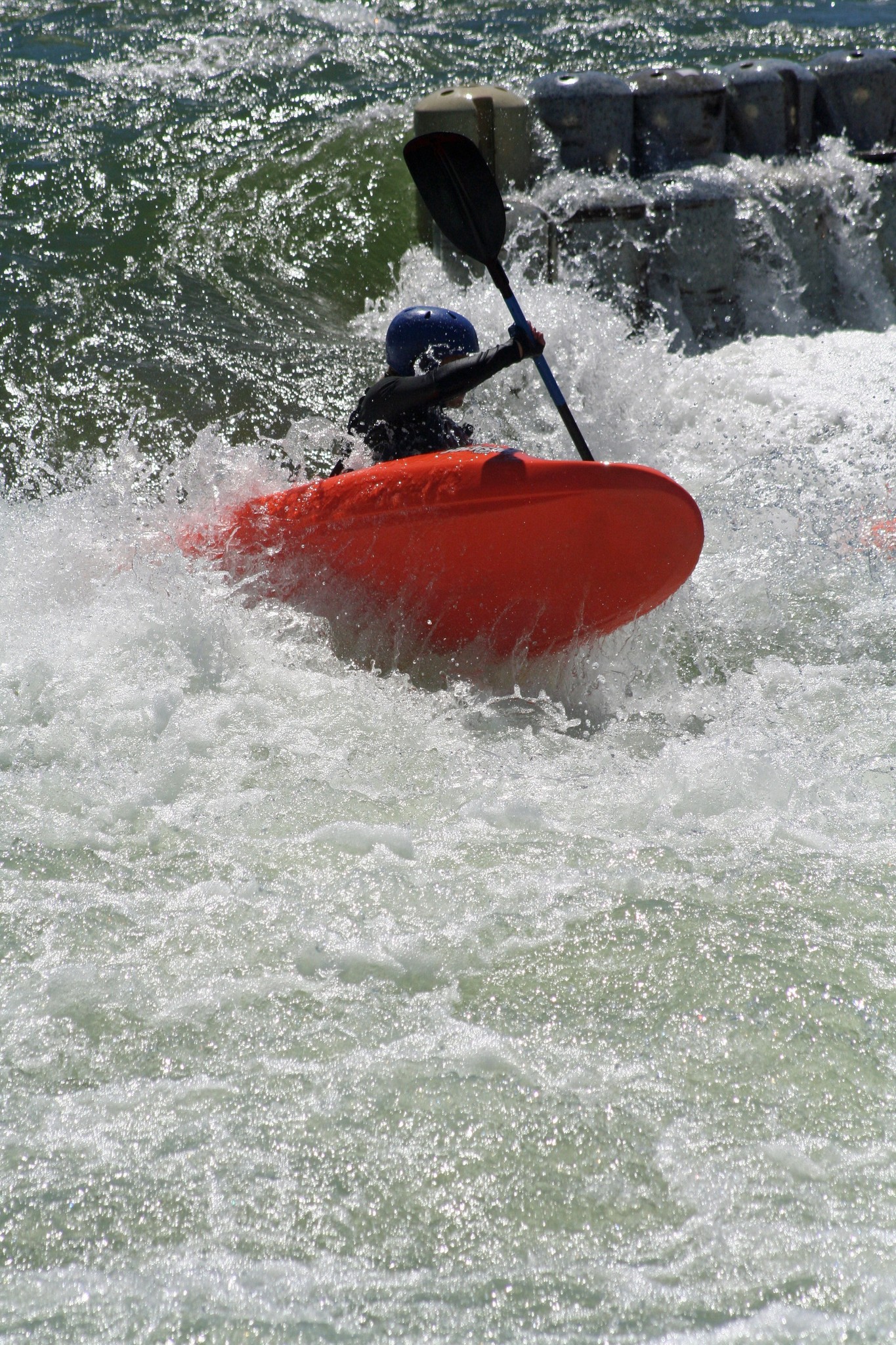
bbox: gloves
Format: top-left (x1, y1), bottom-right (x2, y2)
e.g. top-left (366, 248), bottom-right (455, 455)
top-left (508, 319), bottom-right (547, 359)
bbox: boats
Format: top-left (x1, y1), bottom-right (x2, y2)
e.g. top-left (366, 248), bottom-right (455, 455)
top-left (168, 444), bottom-right (704, 664)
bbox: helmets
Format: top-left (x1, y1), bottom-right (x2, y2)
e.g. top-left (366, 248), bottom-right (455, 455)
top-left (386, 306), bottom-right (480, 378)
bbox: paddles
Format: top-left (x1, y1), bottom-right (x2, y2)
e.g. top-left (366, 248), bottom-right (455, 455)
top-left (401, 129), bottom-right (594, 462)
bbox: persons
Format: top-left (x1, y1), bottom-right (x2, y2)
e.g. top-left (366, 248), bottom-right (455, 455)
top-left (328, 304), bottom-right (546, 479)
